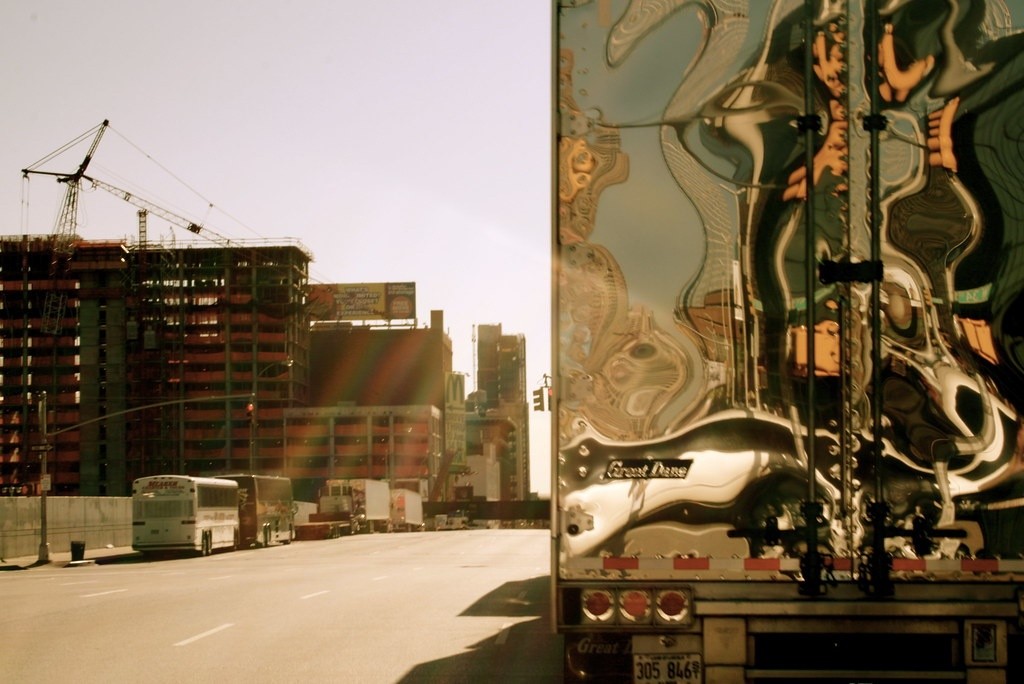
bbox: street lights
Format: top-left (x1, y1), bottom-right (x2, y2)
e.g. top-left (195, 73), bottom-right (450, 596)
top-left (247, 359), bottom-right (294, 473)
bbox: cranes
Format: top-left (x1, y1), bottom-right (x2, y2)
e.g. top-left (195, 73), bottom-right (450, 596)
top-left (20, 118), bottom-right (288, 332)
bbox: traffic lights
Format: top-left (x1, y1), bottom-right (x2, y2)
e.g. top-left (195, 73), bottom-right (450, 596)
top-left (548, 387), bottom-right (553, 410)
top-left (533, 387), bottom-right (544, 411)
top-left (245, 402), bottom-right (254, 424)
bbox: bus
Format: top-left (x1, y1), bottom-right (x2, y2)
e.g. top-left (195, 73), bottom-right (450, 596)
top-left (128, 474), bottom-right (241, 557)
top-left (212, 475), bottom-right (298, 547)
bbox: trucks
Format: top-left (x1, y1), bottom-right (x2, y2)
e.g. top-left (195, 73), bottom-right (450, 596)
top-left (550, 2), bottom-right (1021, 684)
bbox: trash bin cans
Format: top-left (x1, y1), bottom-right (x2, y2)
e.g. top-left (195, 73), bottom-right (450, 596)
top-left (71, 541), bottom-right (86, 561)
